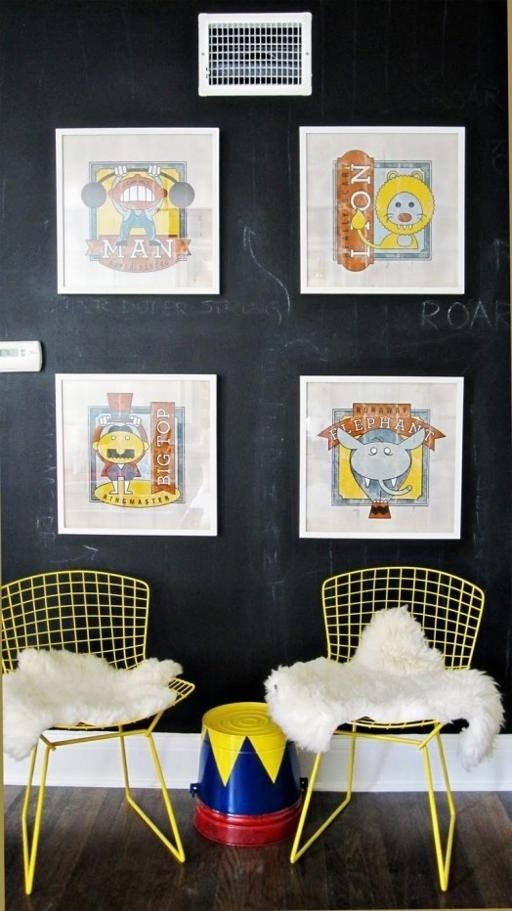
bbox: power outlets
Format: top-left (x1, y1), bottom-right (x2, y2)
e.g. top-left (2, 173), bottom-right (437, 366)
top-left (0, 341), bottom-right (42, 372)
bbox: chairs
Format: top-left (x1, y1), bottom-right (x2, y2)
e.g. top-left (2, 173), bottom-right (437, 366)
top-left (0, 570), bottom-right (196, 896)
top-left (290, 565), bottom-right (485, 892)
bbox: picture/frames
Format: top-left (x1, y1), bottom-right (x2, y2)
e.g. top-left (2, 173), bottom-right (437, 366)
top-left (299, 126), bottom-right (465, 295)
top-left (299, 375), bottom-right (464, 540)
top-left (55, 373), bottom-right (218, 537)
top-left (56, 127), bottom-right (220, 295)
